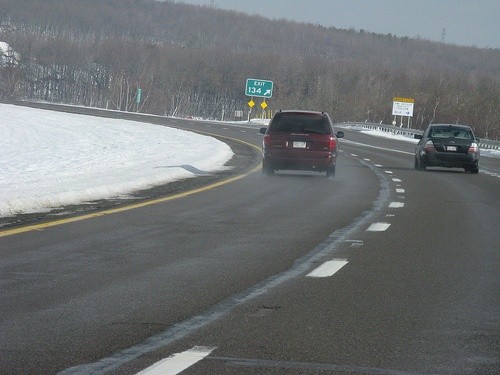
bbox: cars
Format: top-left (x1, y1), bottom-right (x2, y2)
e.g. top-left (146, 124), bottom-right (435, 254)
top-left (412, 123), bottom-right (479, 174)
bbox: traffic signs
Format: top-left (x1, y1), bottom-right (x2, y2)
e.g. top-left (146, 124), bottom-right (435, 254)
top-left (245, 79), bottom-right (273, 98)
top-left (392, 97), bottom-right (414, 117)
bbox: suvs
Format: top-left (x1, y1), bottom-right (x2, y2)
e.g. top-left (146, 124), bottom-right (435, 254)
top-left (261, 110), bottom-right (346, 178)
